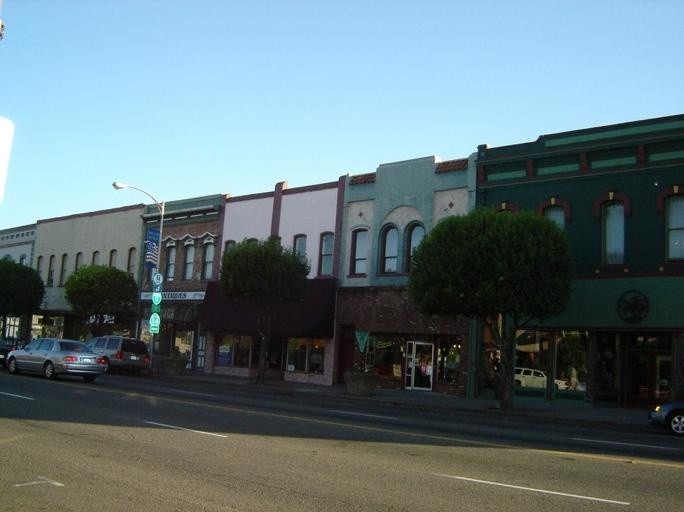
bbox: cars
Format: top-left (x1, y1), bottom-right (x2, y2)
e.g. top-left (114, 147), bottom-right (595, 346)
top-left (650, 399), bottom-right (684, 435)
top-left (6, 337), bottom-right (109, 383)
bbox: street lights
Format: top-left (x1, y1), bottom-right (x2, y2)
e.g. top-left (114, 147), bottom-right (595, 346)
top-left (112, 179), bottom-right (168, 350)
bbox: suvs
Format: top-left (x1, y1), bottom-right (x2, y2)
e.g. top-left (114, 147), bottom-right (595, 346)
top-left (82, 334), bottom-right (152, 376)
top-left (513, 367), bottom-right (570, 391)
